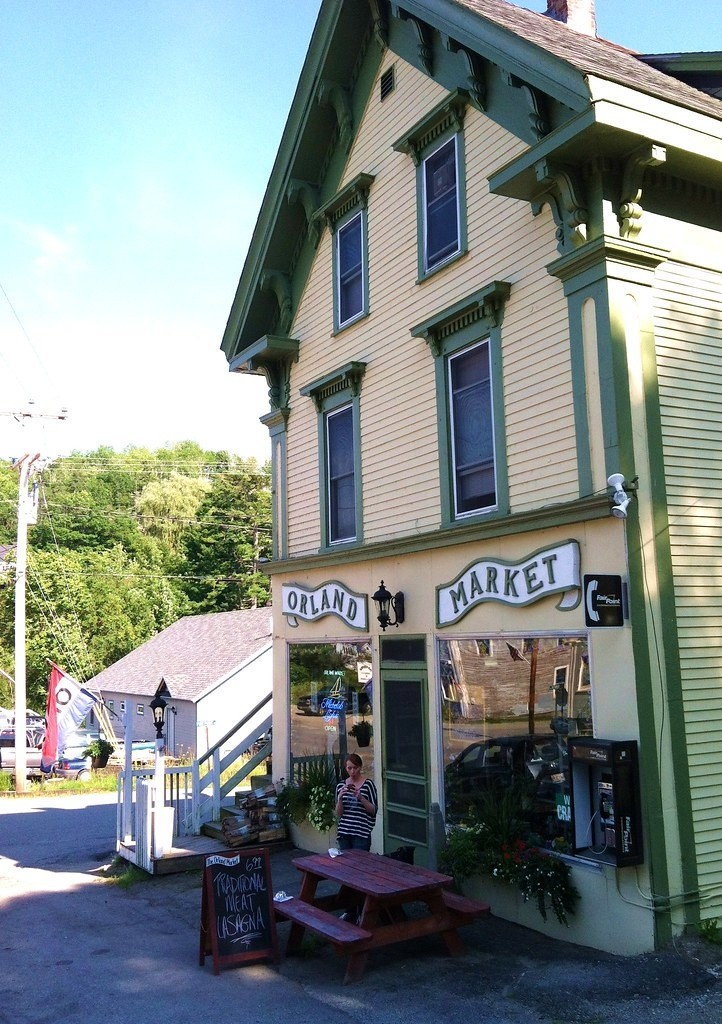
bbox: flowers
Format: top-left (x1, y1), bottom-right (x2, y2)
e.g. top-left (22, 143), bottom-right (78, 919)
top-left (275, 754), bottom-right (334, 832)
top-left (483, 813), bottom-right (582, 921)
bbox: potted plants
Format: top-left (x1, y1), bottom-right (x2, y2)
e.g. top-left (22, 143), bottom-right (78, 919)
top-left (84, 739), bottom-right (114, 768)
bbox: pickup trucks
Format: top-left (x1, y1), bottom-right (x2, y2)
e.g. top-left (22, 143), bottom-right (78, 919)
top-left (298, 685), bottom-right (371, 716)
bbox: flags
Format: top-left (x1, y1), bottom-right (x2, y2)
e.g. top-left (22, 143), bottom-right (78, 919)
top-left (40, 663), bottom-right (98, 773)
top-left (507, 643), bottom-right (523, 661)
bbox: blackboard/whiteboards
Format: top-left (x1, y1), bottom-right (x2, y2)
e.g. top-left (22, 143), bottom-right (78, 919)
top-left (200, 848), bottom-right (279, 964)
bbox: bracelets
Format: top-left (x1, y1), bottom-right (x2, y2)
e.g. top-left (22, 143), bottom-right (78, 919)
top-left (361, 798), bottom-right (365, 801)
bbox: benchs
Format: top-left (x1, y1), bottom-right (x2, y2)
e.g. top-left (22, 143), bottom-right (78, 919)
top-left (423, 889), bottom-right (491, 916)
top-left (272, 895), bottom-right (373, 946)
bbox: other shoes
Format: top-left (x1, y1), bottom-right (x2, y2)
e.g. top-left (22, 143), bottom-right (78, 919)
top-left (338, 912), bottom-right (358, 923)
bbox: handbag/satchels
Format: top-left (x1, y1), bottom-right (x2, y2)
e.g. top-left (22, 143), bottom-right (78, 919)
top-left (382, 846), bottom-right (416, 866)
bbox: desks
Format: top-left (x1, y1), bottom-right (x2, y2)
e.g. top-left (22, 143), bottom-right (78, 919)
top-left (282, 849), bottom-right (468, 986)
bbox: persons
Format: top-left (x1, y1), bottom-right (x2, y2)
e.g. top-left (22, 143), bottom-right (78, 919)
top-left (335, 754), bottom-right (379, 922)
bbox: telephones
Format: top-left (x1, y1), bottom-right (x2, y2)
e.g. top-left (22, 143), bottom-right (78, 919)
top-left (599, 789), bottom-right (614, 823)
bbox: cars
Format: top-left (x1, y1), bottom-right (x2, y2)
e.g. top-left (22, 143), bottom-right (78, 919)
top-left (0, 709), bottom-right (156, 782)
top-left (444, 734), bottom-right (569, 812)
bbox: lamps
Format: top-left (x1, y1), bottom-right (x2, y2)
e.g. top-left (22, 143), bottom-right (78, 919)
top-left (610, 498), bottom-right (631, 518)
top-left (606, 471), bottom-right (628, 503)
top-left (171, 706), bottom-right (177, 715)
top-left (371, 580), bottom-right (405, 632)
